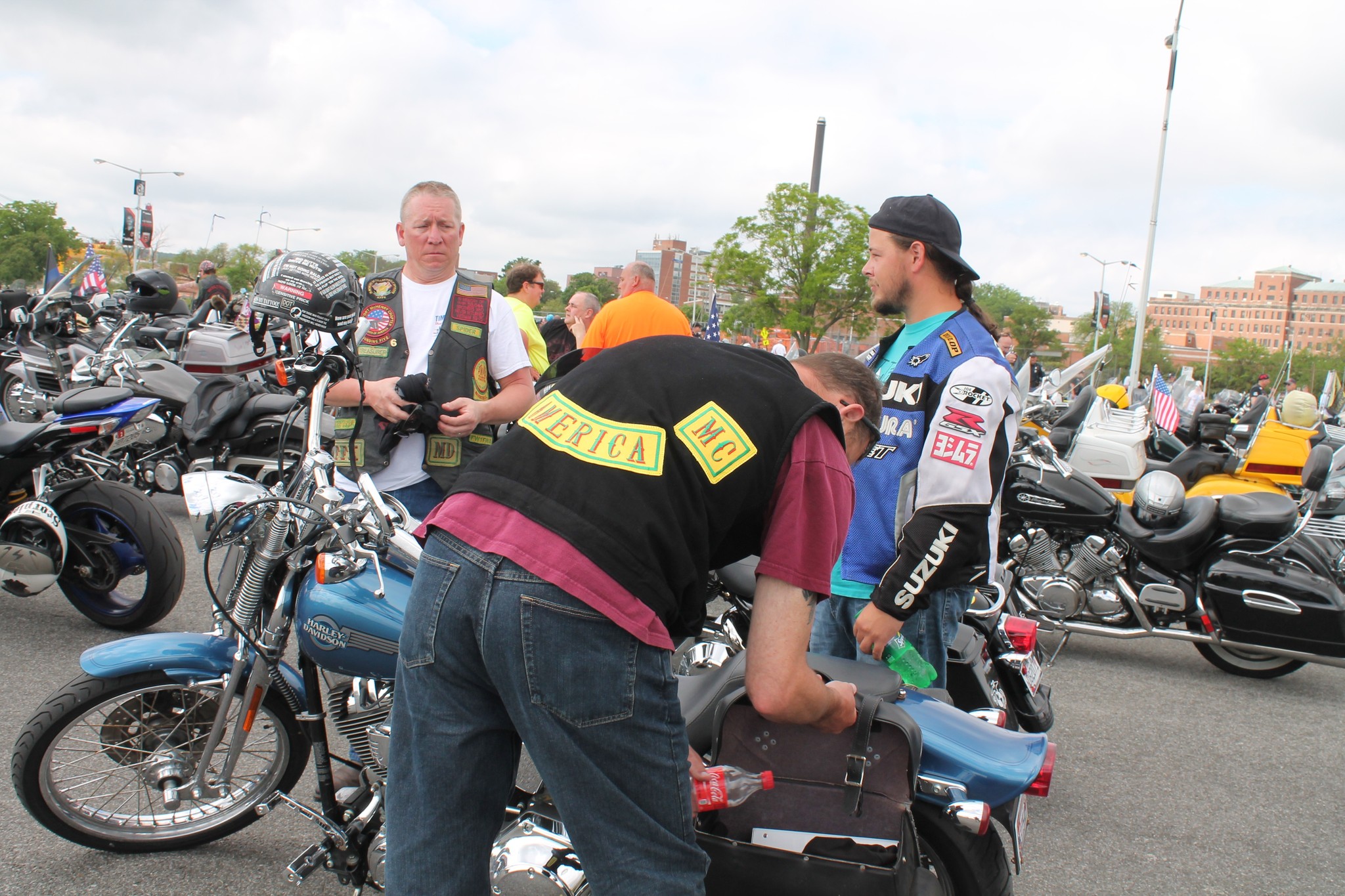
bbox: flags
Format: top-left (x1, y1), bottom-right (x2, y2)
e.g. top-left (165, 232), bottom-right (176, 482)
top-left (44, 247), bottom-right (62, 293)
top-left (78, 244), bottom-right (107, 297)
top-left (704, 294), bottom-right (720, 342)
top-left (1148, 367), bottom-right (1180, 432)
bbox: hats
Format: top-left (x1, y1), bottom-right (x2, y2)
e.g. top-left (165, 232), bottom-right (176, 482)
top-left (1258, 374), bottom-right (1270, 380)
top-left (868, 193), bottom-right (980, 281)
top-left (1283, 379), bottom-right (1297, 385)
top-left (1028, 352), bottom-right (1037, 357)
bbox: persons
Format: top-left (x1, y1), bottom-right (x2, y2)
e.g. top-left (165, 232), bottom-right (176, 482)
top-left (581, 261), bottom-right (692, 349)
top-left (999, 332), bottom-right (1308, 418)
top-left (307, 180), bottom-right (536, 519)
top-left (177, 261), bottom-right (231, 313)
top-left (691, 322), bottom-right (808, 358)
top-left (504, 264), bottom-right (549, 387)
top-left (807, 195), bottom-right (1023, 689)
top-left (540, 292), bottom-right (600, 363)
top-left (386, 336), bottom-right (884, 896)
top-left (539, 314), bottom-right (561, 325)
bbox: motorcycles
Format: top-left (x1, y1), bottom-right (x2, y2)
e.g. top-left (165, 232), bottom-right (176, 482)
top-left (0, 267), bottom-right (1345, 675)
top-left (6, 253), bottom-right (1060, 896)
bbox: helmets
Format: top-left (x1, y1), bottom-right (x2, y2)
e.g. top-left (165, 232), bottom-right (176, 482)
top-left (250, 249), bottom-right (362, 333)
top-left (199, 260), bottom-right (215, 272)
top-left (124, 269), bottom-right (178, 314)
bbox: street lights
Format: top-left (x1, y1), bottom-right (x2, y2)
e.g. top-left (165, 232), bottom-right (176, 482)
top-left (257, 220), bottom-right (320, 254)
top-left (1081, 253), bottom-right (1127, 391)
top-left (1285, 327), bottom-right (1305, 398)
top-left (1201, 299), bottom-right (1229, 398)
top-left (94, 158), bottom-right (184, 272)
top-left (672, 247), bottom-right (713, 325)
top-left (353, 249), bottom-right (400, 274)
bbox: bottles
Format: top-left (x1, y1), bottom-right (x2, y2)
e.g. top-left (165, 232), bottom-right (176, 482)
top-left (855, 608), bottom-right (938, 689)
top-left (689, 764), bottom-right (775, 813)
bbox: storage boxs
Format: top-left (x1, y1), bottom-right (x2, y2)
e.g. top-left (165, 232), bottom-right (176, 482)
top-left (692, 686), bottom-right (923, 896)
top-left (1197, 413), bottom-right (1231, 440)
top-left (1202, 553), bottom-right (1345, 657)
top-left (183, 319), bottom-right (276, 380)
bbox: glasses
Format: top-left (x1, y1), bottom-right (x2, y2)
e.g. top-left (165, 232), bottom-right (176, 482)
top-left (840, 399), bottom-right (882, 461)
top-left (1000, 332), bottom-right (1012, 338)
top-left (522, 281), bottom-right (544, 290)
